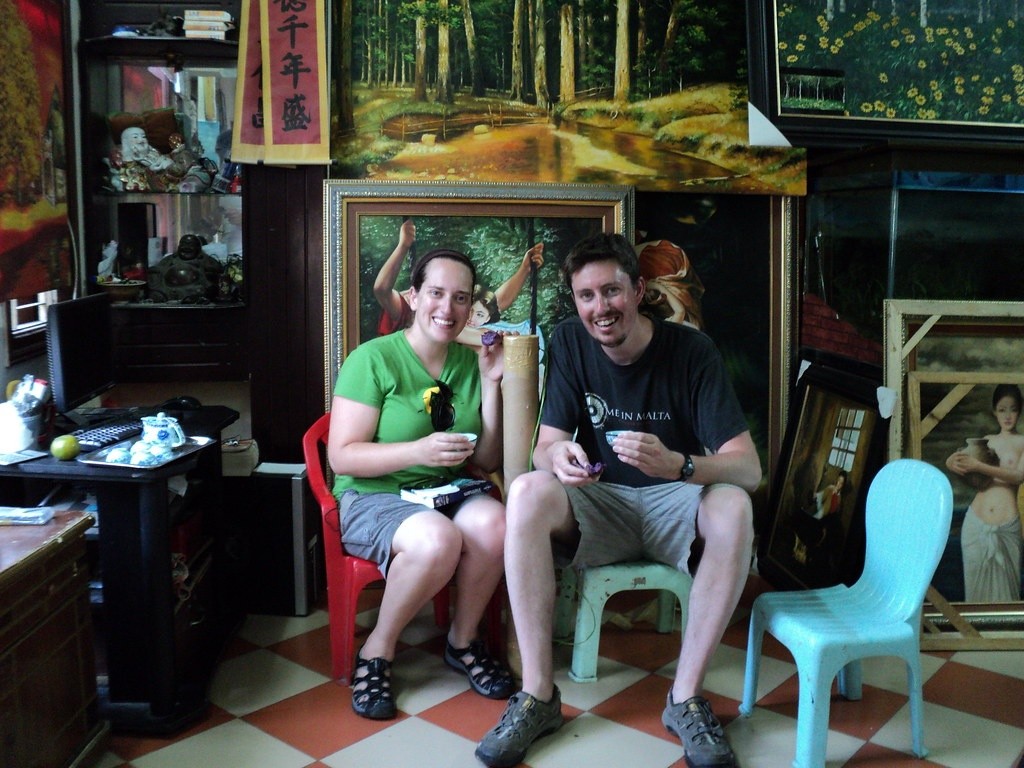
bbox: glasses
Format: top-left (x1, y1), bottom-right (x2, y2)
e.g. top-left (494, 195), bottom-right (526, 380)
top-left (430, 379), bottom-right (457, 435)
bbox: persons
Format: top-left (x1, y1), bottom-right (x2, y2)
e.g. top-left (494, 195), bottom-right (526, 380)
top-left (475, 233), bottom-right (762, 768)
top-left (108, 128), bottom-right (209, 193)
top-left (327, 248), bottom-right (520, 721)
top-left (147, 235), bottom-right (225, 300)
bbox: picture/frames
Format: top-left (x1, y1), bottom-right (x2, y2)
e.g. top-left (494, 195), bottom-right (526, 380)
top-left (884, 297), bottom-right (1024, 628)
top-left (323, 179), bottom-right (637, 588)
top-left (635, 193), bottom-right (792, 544)
top-left (909, 371), bottom-right (1024, 652)
top-left (758, 346), bottom-right (883, 591)
top-left (746, 0), bottom-right (1024, 150)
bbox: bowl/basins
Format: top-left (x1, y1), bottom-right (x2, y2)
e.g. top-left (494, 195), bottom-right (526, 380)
top-left (100, 280), bottom-right (147, 302)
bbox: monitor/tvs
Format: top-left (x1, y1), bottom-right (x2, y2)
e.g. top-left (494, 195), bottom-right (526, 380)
top-left (47, 292), bottom-right (123, 414)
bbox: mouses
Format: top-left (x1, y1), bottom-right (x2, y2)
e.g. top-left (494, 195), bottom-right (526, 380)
top-left (162, 396), bottom-right (201, 411)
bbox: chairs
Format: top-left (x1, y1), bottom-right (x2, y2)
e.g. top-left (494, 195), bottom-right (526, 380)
top-left (739, 459), bottom-right (954, 768)
top-left (303, 411), bottom-right (508, 689)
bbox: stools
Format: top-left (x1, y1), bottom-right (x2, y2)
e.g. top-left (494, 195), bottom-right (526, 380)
top-left (555, 560), bottom-right (694, 683)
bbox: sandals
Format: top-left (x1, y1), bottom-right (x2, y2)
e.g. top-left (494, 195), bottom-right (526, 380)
top-left (444, 637), bottom-right (517, 699)
top-left (351, 644), bottom-right (396, 721)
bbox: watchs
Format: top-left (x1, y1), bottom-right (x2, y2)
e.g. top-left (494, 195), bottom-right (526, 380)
top-left (673, 453), bottom-right (695, 482)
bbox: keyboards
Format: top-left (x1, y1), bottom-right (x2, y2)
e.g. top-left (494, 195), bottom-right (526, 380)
top-left (68, 407), bottom-right (162, 450)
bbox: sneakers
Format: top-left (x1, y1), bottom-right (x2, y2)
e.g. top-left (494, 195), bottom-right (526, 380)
top-left (662, 688), bottom-right (737, 768)
top-left (475, 682), bottom-right (564, 768)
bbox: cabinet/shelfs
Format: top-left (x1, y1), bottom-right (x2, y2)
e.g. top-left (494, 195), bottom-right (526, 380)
top-left (65, 0), bottom-right (243, 314)
top-left (0, 403), bottom-right (239, 738)
top-left (0, 510), bottom-right (110, 768)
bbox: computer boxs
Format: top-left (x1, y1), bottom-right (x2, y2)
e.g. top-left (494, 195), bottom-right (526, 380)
top-left (214, 462), bottom-right (317, 618)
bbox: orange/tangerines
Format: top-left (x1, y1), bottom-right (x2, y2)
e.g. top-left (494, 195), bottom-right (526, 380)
top-left (50, 435), bottom-right (80, 460)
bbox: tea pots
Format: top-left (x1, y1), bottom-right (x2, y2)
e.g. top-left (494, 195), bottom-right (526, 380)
top-left (140, 412), bottom-right (186, 448)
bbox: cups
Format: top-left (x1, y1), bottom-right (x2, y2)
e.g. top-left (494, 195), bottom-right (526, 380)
top-left (455, 432), bottom-right (478, 452)
top-left (105, 440), bottom-right (172, 466)
top-left (605, 430), bottom-right (629, 446)
top-left (20, 402), bottom-right (59, 452)
